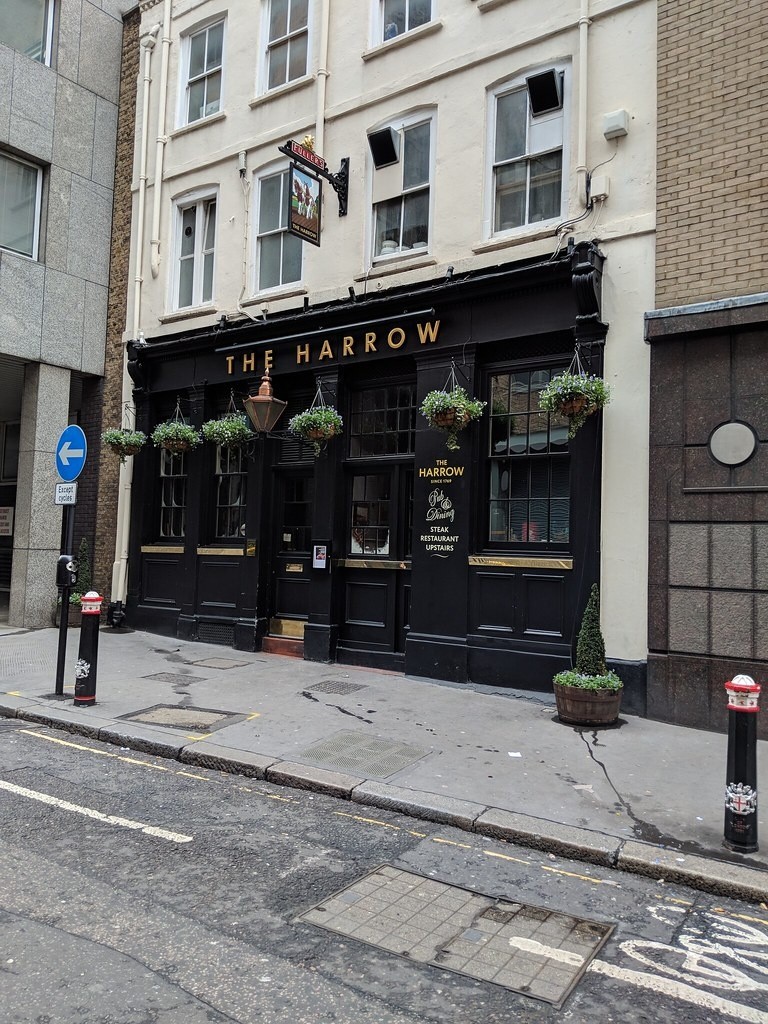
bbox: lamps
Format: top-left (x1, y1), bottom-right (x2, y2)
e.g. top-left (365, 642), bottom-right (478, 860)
top-left (81, 591), bottom-right (103, 614)
top-left (724, 674), bottom-right (762, 713)
top-left (242, 367), bottom-right (288, 435)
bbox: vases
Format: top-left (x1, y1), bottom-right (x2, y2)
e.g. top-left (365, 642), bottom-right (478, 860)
top-left (554, 392), bottom-right (601, 422)
top-left (551, 681), bottom-right (623, 725)
top-left (430, 407), bottom-right (473, 434)
top-left (306, 423), bottom-right (335, 445)
top-left (160, 438), bottom-right (194, 456)
top-left (109, 442), bottom-right (143, 456)
top-left (56, 604), bottom-right (83, 627)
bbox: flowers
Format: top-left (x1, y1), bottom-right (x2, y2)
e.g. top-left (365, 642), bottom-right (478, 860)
top-left (491, 398), bottom-right (521, 455)
top-left (57, 536), bottom-right (91, 607)
top-left (288, 404), bottom-right (344, 458)
top-left (552, 583), bottom-right (624, 692)
top-left (99, 426), bottom-right (148, 469)
top-left (199, 414), bottom-right (255, 451)
top-left (536, 371), bottom-right (617, 439)
top-left (419, 384), bottom-right (489, 452)
top-left (150, 421), bottom-right (203, 452)
